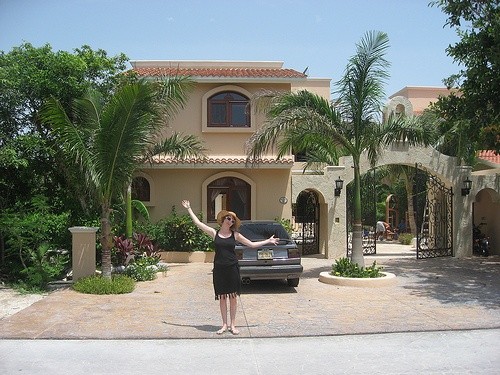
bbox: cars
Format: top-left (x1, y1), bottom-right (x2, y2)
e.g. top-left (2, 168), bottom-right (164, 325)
top-left (233, 220), bottom-right (303, 287)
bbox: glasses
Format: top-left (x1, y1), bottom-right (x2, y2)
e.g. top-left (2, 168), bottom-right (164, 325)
top-left (226, 217), bottom-right (235, 224)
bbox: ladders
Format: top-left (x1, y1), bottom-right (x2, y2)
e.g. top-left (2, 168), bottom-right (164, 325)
top-left (420, 196), bottom-right (429, 249)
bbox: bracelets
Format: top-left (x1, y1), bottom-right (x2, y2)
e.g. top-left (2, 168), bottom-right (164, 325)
top-left (186, 206), bottom-right (190, 210)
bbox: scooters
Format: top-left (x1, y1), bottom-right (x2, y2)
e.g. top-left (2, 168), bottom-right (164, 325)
top-left (473, 216), bottom-right (489, 257)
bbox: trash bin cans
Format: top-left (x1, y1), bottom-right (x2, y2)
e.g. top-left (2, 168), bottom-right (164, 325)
top-left (365, 231), bottom-right (368, 235)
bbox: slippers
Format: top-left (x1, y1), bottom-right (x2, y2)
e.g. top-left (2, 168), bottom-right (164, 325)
top-left (216, 326), bottom-right (226, 334)
top-left (228, 327), bottom-right (239, 335)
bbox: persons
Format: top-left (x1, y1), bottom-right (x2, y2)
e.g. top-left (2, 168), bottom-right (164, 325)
top-left (375, 220), bottom-right (391, 240)
top-left (181, 199), bottom-right (280, 335)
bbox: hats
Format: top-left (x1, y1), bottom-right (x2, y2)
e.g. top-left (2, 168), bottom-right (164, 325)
top-left (217, 210), bottom-right (241, 232)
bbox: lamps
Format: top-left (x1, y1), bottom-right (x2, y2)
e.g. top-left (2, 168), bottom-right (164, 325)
top-left (334, 176), bottom-right (343, 196)
top-left (461, 177), bottom-right (472, 196)
